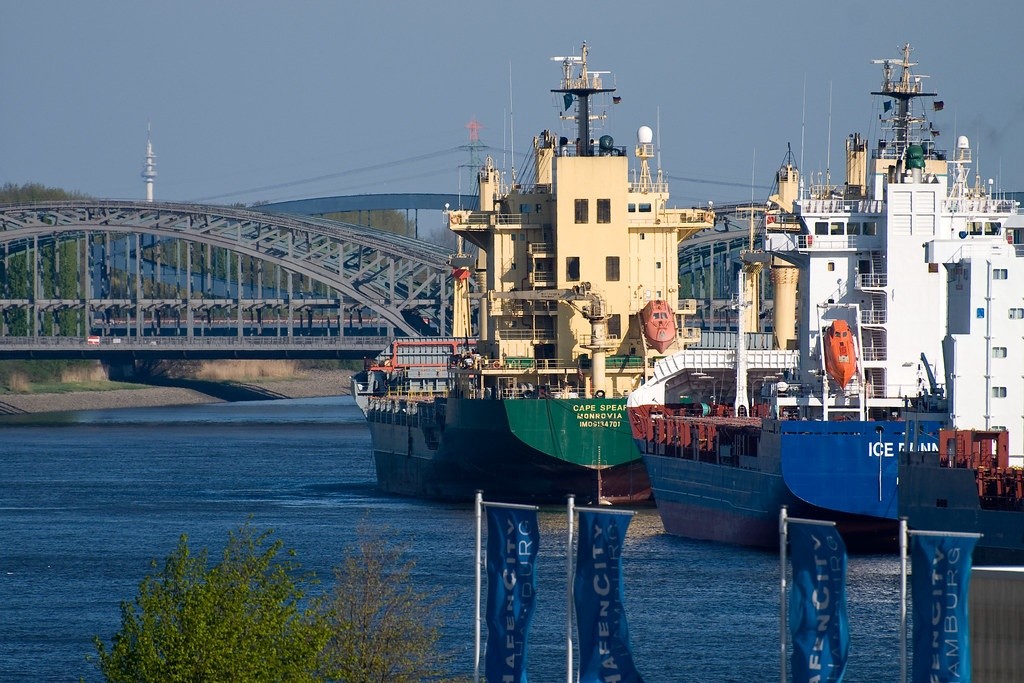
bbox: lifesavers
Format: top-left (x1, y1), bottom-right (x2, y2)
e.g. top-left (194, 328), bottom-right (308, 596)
top-left (493, 360), bottom-right (501, 370)
top-left (451, 215), bottom-right (459, 225)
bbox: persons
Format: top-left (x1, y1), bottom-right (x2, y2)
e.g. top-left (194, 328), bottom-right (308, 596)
top-left (463, 356), bottom-right (473, 371)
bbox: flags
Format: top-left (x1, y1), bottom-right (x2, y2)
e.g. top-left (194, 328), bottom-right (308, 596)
top-left (884, 100), bottom-right (892, 113)
top-left (934, 100), bottom-right (945, 112)
top-left (481, 501), bottom-right (540, 683)
top-left (909, 530), bottom-right (983, 683)
top-left (931, 130), bottom-right (939, 137)
top-left (578, 364), bottom-right (585, 381)
top-left (563, 94), bottom-right (572, 111)
top-left (613, 95), bottom-right (621, 104)
top-left (573, 507), bottom-right (645, 682)
top-left (785, 519), bottom-right (850, 683)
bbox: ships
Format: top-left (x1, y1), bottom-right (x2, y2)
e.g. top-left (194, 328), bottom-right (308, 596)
top-left (351, 40), bottom-right (717, 509)
top-left (625, 41), bottom-right (1024, 566)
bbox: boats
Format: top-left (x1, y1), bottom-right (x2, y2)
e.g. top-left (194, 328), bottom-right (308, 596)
top-left (823, 318), bottom-right (857, 388)
top-left (641, 299), bottom-right (677, 354)
